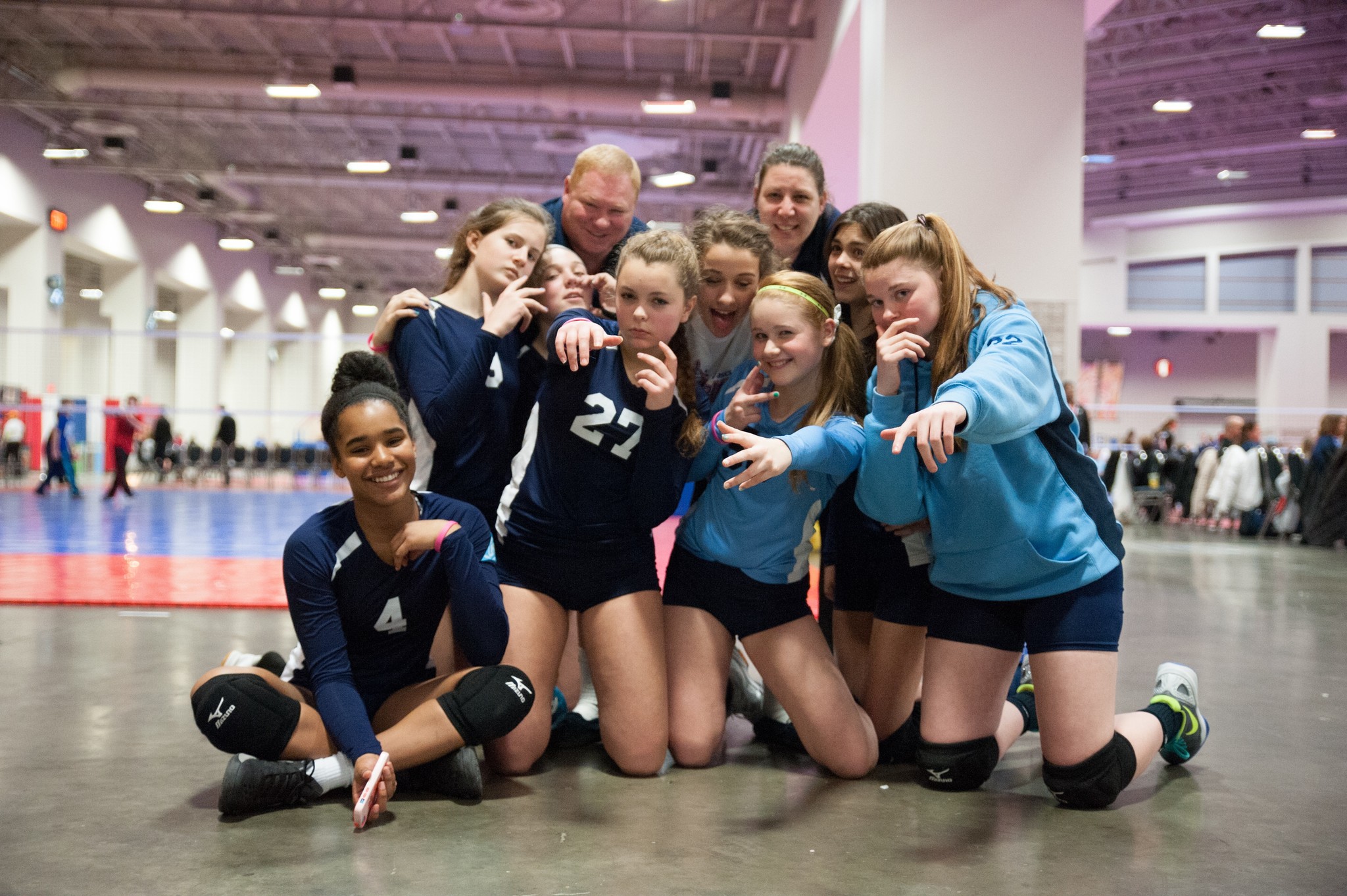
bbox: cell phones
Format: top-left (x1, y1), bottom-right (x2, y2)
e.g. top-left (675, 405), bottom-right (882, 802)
top-left (354, 750), bottom-right (392, 828)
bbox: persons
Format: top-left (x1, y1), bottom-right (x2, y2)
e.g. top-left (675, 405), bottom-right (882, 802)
top-left (0, 398), bottom-right (237, 507)
top-left (191, 145), bottom-right (1213, 820)
top-left (1109, 414), bottom-right (1347, 552)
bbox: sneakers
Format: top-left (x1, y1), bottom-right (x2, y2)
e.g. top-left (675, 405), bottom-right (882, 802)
top-left (1154, 662), bottom-right (1210, 766)
top-left (399, 746), bottom-right (485, 805)
top-left (1016, 664), bottom-right (1035, 692)
top-left (724, 638), bottom-right (793, 724)
top-left (218, 752), bottom-right (324, 813)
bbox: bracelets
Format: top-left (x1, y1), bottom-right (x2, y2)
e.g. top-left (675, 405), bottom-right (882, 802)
top-left (555, 317), bottom-right (593, 355)
top-left (368, 331), bottom-right (391, 352)
top-left (434, 521), bottom-right (459, 553)
top-left (712, 409), bottom-right (733, 447)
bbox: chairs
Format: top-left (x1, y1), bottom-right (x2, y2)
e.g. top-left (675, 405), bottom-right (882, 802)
top-left (139, 440), bottom-right (335, 489)
top-left (1128, 443), bottom-right (1347, 546)
